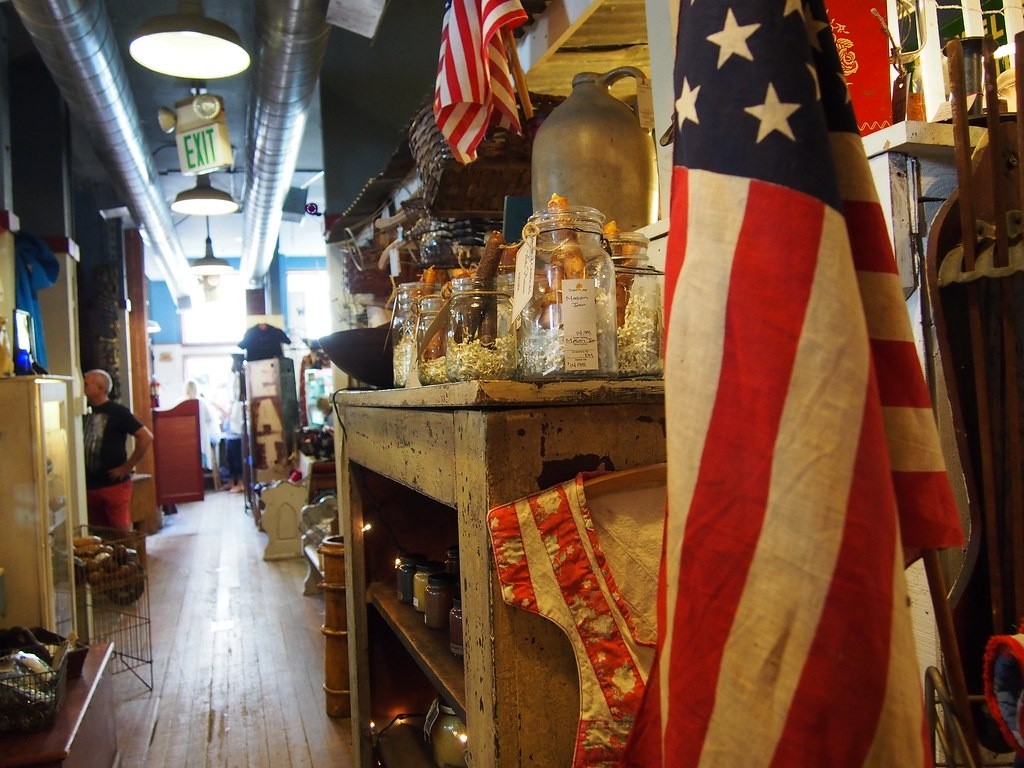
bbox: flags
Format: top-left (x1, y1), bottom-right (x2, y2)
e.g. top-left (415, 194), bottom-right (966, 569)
top-left (620, 2), bottom-right (966, 766)
top-left (433, 1), bottom-right (534, 166)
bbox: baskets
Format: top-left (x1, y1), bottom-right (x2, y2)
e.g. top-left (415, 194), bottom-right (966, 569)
top-left (406, 91), bottom-right (567, 220)
top-left (1, 639), bottom-right (66, 736)
top-left (50, 523), bottom-right (153, 704)
top-left (344, 243), bottom-right (426, 295)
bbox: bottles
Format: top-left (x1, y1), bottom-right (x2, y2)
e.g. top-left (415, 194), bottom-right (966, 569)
top-left (421, 571), bottom-right (461, 629)
top-left (391, 281), bottom-right (443, 388)
top-left (447, 594), bottom-right (464, 661)
top-left (448, 276), bottom-right (518, 383)
top-left (395, 554), bottom-right (429, 603)
top-left (416, 294), bottom-right (450, 386)
top-left (411, 561), bottom-right (441, 613)
top-left (511, 201), bottom-right (619, 382)
top-left (606, 227), bottom-right (666, 382)
top-left (529, 65), bottom-right (660, 234)
top-left (439, 546), bottom-right (460, 578)
top-left (430, 701), bottom-right (469, 768)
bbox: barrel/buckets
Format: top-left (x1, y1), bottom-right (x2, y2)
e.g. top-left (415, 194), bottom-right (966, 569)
top-left (316, 534), bottom-right (353, 717)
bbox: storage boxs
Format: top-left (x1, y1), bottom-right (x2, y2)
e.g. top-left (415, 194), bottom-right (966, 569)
top-left (407, 91), bottom-right (569, 219)
top-left (0, 639), bottom-right (70, 733)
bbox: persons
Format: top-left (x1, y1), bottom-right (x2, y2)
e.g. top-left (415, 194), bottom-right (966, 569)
top-left (183, 382), bottom-right (221, 472)
top-left (82, 369), bottom-right (154, 531)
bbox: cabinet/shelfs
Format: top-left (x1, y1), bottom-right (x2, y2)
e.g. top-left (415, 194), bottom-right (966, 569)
top-left (327, 375), bottom-right (666, 768)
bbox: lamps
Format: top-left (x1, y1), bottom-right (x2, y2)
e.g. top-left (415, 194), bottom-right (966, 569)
top-left (171, 174), bottom-right (239, 215)
top-left (129, 0), bottom-right (251, 80)
top-left (190, 214), bottom-right (236, 277)
